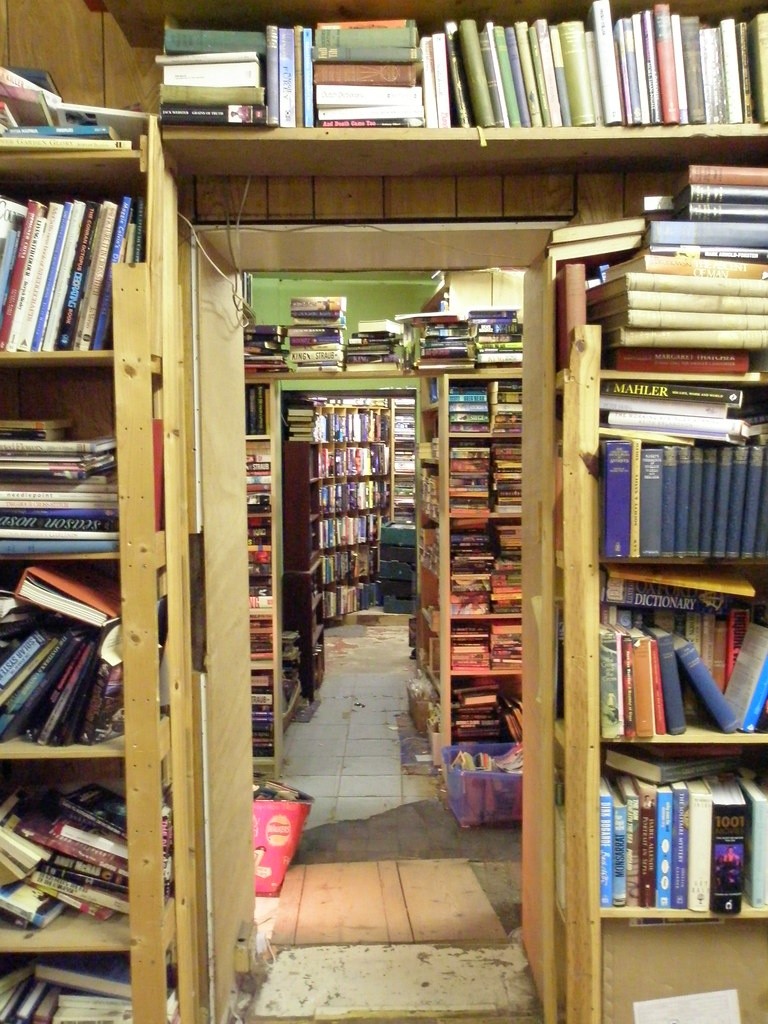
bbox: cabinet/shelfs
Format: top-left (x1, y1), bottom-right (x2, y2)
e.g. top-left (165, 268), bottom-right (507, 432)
top-left (0, 0), bottom-right (767, 1024)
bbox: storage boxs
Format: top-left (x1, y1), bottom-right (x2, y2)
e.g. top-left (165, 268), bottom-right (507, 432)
top-left (252, 780), bottom-right (314, 893)
top-left (441, 743), bottom-right (522, 829)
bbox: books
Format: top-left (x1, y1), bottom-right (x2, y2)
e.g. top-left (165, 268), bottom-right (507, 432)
top-left (0, 2), bottom-right (768, 1023)
